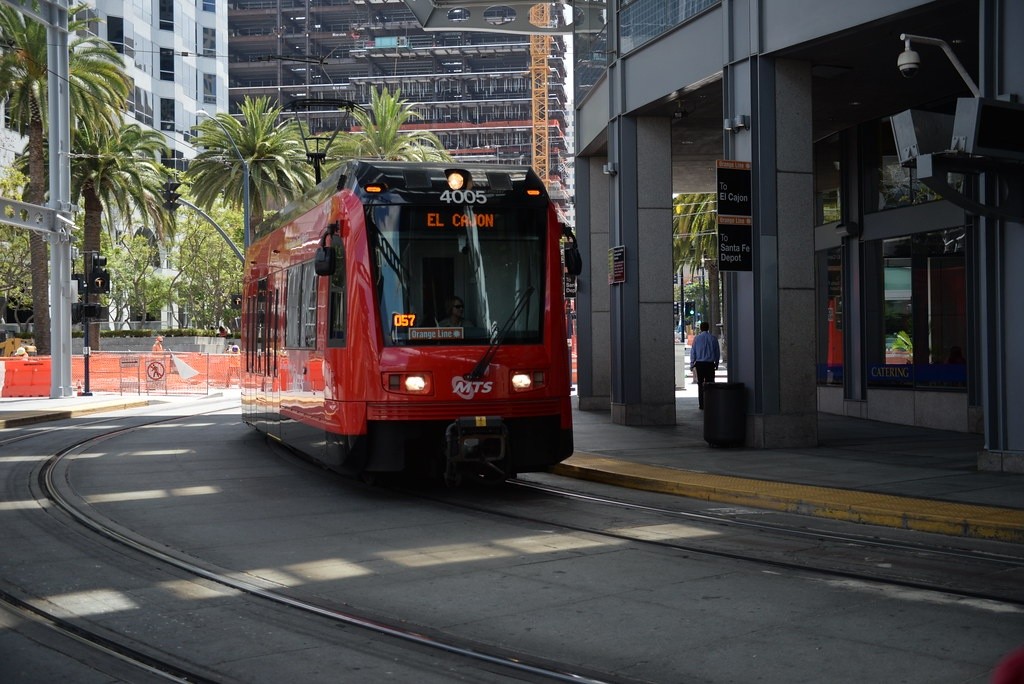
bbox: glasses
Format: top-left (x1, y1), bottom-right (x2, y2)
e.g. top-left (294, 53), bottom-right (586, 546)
top-left (453, 305), bottom-right (464, 309)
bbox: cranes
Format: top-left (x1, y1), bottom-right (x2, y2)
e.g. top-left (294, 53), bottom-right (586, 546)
top-left (526, 4), bottom-right (553, 193)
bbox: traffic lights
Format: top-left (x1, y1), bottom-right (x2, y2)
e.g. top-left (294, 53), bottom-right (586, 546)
top-left (88, 272), bottom-right (110, 293)
top-left (160, 182), bottom-right (181, 212)
top-left (674, 273), bottom-right (678, 284)
top-left (688, 301), bottom-right (695, 315)
top-left (91, 251), bottom-right (107, 274)
top-left (231, 294), bottom-right (242, 310)
top-left (71, 273), bottom-right (85, 294)
top-left (674, 301), bottom-right (679, 316)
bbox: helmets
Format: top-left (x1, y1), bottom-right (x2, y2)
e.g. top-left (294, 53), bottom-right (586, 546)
top-left (156, 336), bottom-right (163, 342)
top-left (17, 347), bottom-right (25, 355)
top-left (232, 345), bottom-right (238, 352)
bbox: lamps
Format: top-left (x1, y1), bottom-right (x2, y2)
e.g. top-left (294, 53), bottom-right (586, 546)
top-left (723, 115), bottom-right (750, 135)
top-left (602, 162), bottom-right (618, 178)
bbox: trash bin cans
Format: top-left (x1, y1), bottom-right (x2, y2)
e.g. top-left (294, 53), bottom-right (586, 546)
top-left (703, 382), bottom-right (747, 449)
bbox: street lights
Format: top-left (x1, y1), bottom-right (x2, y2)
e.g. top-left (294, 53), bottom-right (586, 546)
top-left (194, 110), bottom-right (251, 259)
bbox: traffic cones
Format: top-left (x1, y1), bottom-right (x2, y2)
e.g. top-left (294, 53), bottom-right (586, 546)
top-left (76, 379), bottom-right (84, 397)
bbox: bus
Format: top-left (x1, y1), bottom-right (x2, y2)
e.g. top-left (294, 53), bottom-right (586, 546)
top-left (241, 100), bottom-right (584, 490)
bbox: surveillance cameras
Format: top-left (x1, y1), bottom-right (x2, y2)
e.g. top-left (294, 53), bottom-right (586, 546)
top-left (897, 51), bottom-right (920, 78)
top-left (55, 214), bottom-right (80, 233)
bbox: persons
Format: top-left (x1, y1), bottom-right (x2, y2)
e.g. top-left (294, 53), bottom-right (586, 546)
top-left (15, 347), bottom-right (28, 358)
top-left (438, 297), bottom-right (474, 334)
top-left (224, 341), bottom-right (241, 388)
top-left (690, 322), bottom-right (721, 409)
top-left (152, 336), bottom-right (163, 355)
